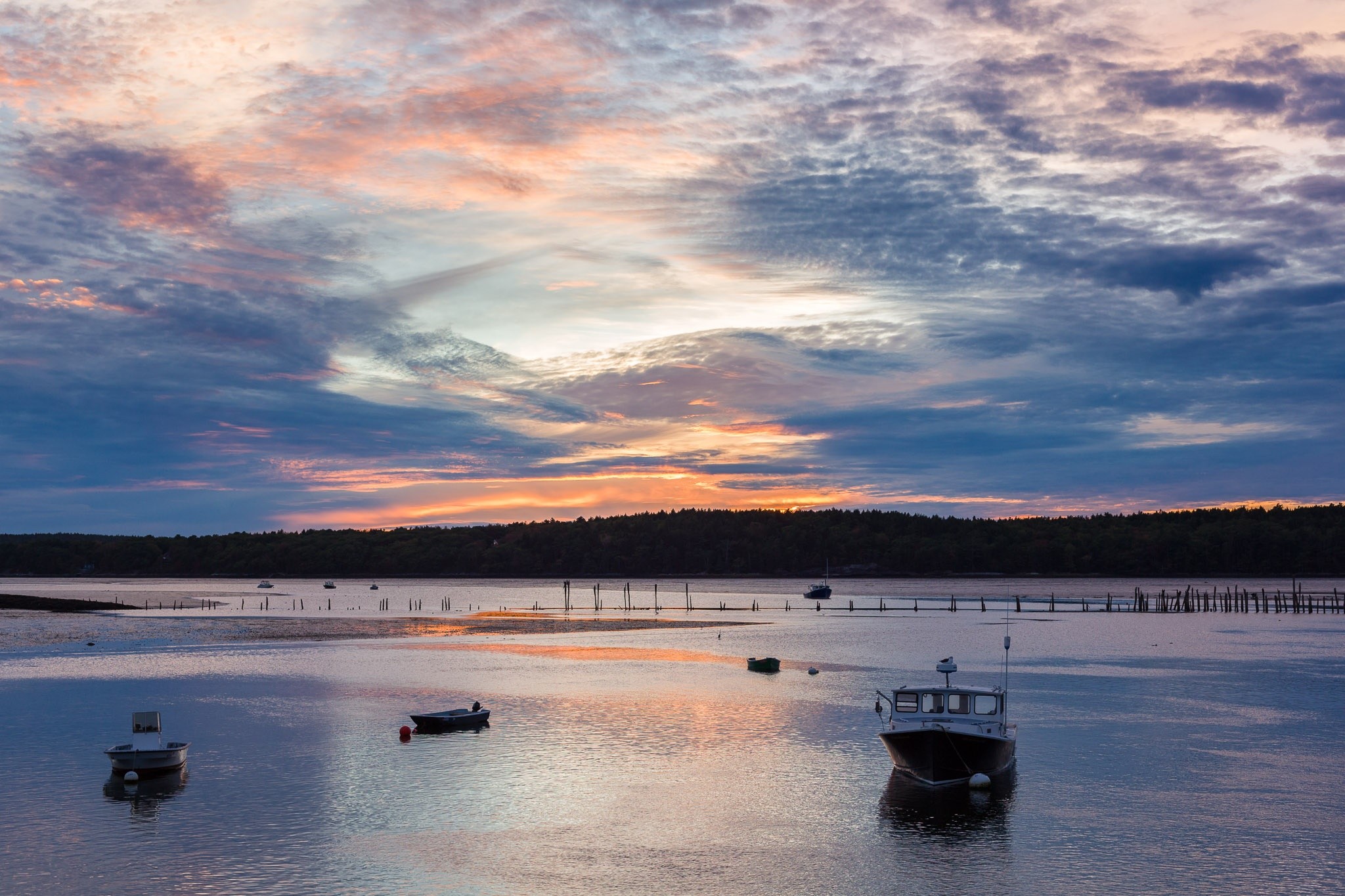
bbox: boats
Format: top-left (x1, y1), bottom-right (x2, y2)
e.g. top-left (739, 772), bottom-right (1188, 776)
top-left (873, 579), bottom-right (1021, 786)
top-left (323, 581), bottom-right (337, 589)
top-left (257, 580), bottom-right (274, 589)
top-left (370, 583), bottom-right (379, 589)
top-left (410, 699), bottom-right (490, 731)
top-left (746, 656), bottom-right (781, 674)
top-left (104, 709), bottom-right (189, 776)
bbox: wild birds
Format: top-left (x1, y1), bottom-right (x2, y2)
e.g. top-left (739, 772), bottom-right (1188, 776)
top-left (808, 667), bottom-right (819, 674)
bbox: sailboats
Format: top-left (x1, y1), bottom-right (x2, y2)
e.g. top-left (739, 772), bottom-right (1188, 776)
top-left (804, 557), bottom-right (833, 600)
